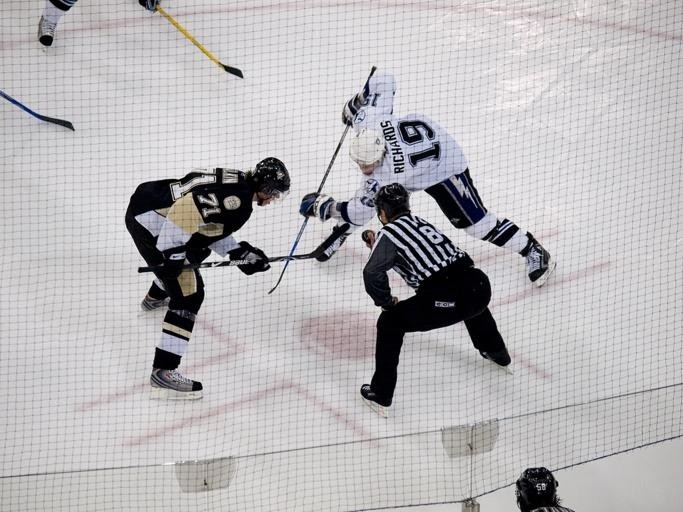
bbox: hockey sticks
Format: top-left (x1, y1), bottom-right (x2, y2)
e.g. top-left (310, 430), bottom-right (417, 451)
top-left (0, 92), bottom-right (74, 132)
top-left (267, 66), bottom-right (379, 294)
top-left (153, 4), bottom-right (244, 80)
top-left (136, 223), bottom-right (351, 273)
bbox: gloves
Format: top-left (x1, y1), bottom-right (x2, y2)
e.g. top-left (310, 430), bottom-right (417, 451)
top-left (163, 244), bottom-right (186, 276)
top-left (229, 240), bottom-right (271, 276)
top-left (139, 0), bottom-right (161, 12)
top-left (342, 93), bottom-right (369, 127)
top-left (382, 296), bottom-right (397, 312)
top-left (300, 193), bottom-right (335, 222)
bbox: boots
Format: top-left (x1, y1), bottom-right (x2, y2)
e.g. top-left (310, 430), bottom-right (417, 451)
top-left (479, 349), bottom-right (512, 366)
top-left (150, 368), bottom-right (203, 393)
top-left (142, 294), bottom-right (170, 310)
top-left (36, 14), bottom-right (56, 46)
top-left (361, 381), bottom-right (392, 406)
top-left (525, 237), bottom-right (550, 282)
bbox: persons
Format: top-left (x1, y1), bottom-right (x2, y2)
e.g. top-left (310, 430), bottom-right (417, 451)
top-left (300, 72), bottom-right (557, 288)
top-left (513, 466), bottom-right (580, 512)
top-left (35, 0), bottom-right (162, 47)
top-left (360, 184), bottom-right (514, 418)
top-left (125, 156), bottom-right (292, 392)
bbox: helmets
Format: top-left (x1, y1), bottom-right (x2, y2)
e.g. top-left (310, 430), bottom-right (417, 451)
top-left (350, 128), bottom-right (384, 166)
top-left (254, 158), bottom-right (290, 203)
top-left (373, 182), bottom-right (408, 221)
top-left (514, 467), bottom-right (559, 512)
